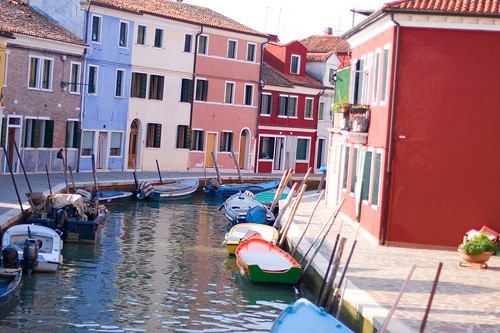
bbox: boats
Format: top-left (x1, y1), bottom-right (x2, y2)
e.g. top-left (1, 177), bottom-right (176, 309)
top-left (0, 257), bottom-right (24, 309)
top-left (235, 239), bottom-right (303, 286)
top-left (1, 137), bottom-right (108, 245)
top-left (221, 222), bottom-right (279, 256)
top-left (222, 189), bottom-right (276, 226)
top-left (132, 159), bottom-right (200, 202)
top-left (201, 150), bottom-right (280, 199)
top-left (270, 298), bottom-right (355, 333)
top-left (56, 148), bottom-right (134, 206)
top-left (251, 185), bottom-right (292, 211)
top-left (2, 222), bottom-right (63, 274)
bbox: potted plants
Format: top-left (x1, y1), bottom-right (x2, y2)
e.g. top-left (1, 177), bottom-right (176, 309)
top-left (332, 103), bottom-right (341, 112)
top-left (456, 228), bottom-right (497, 264)
top-left (340, 95), bottom-right (349, 113)
top-left (351, 104), bottom-right (368, 114)
